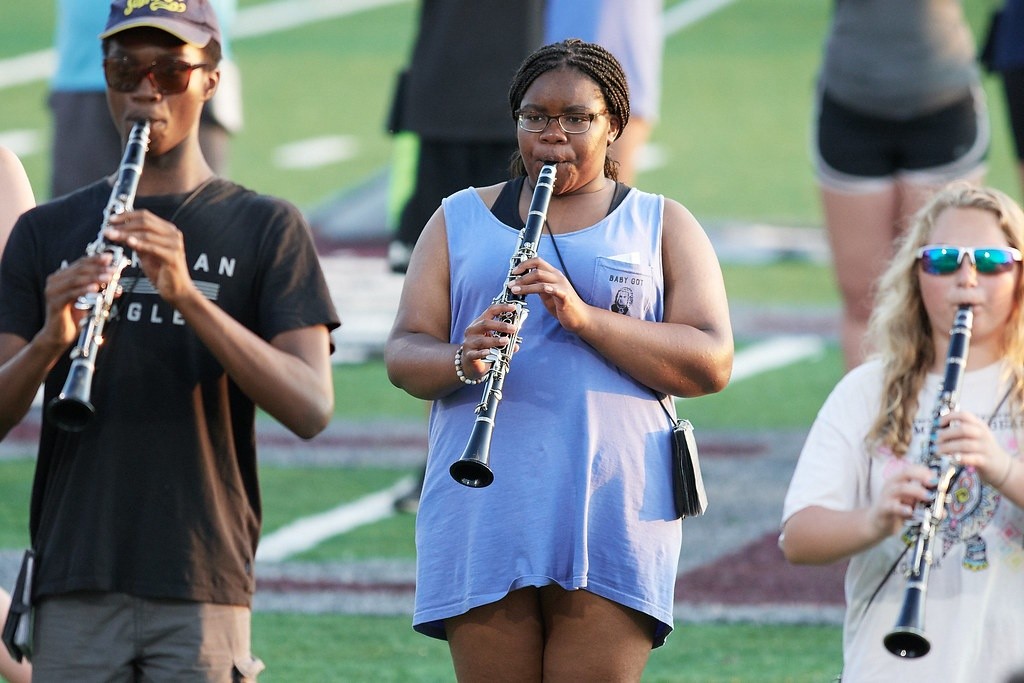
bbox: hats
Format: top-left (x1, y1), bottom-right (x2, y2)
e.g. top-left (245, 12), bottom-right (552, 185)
top-left (97, 0), bottom-right (222, 48)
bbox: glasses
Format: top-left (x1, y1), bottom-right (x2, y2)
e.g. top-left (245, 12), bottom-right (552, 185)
top-left (102, 56), bottom-right (209, 96)
top-left (515, 108), bottom-right (609, 135)
top-left (916, 243), bottom-right (1023, 276)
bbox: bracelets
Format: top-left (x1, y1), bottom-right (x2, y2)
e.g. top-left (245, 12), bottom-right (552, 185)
top-left (993, 454), bottom-right (1014, 489)
top-left (454, 345), bottom-right (491, 385)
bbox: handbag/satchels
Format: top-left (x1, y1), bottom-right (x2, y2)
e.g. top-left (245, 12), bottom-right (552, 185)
top-left (671, 418), bottom-right (709, 519)
top-left (1, 549), bottom-right (40, 665)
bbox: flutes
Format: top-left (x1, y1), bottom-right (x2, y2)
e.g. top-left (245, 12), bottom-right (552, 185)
top-left (49, 119), bottom-right (153, 431)
top-left (884, 305), bottom-right (973, 657)
top-left (449, 160), bottom-right (556, 489)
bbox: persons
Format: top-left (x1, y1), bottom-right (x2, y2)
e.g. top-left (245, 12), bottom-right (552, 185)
top-left (812, 2), bottom-right (994, 369)
top-left (390, 0), bottom-right (664, 269)
top-left (777, 176), bottom-right (1023, 683)
top-left (385, 40), bottom-right (735, 681)
top-left (48, 0), bottom-right (239, 198)
top-left (1, 0), bottom-right (340, 683)
